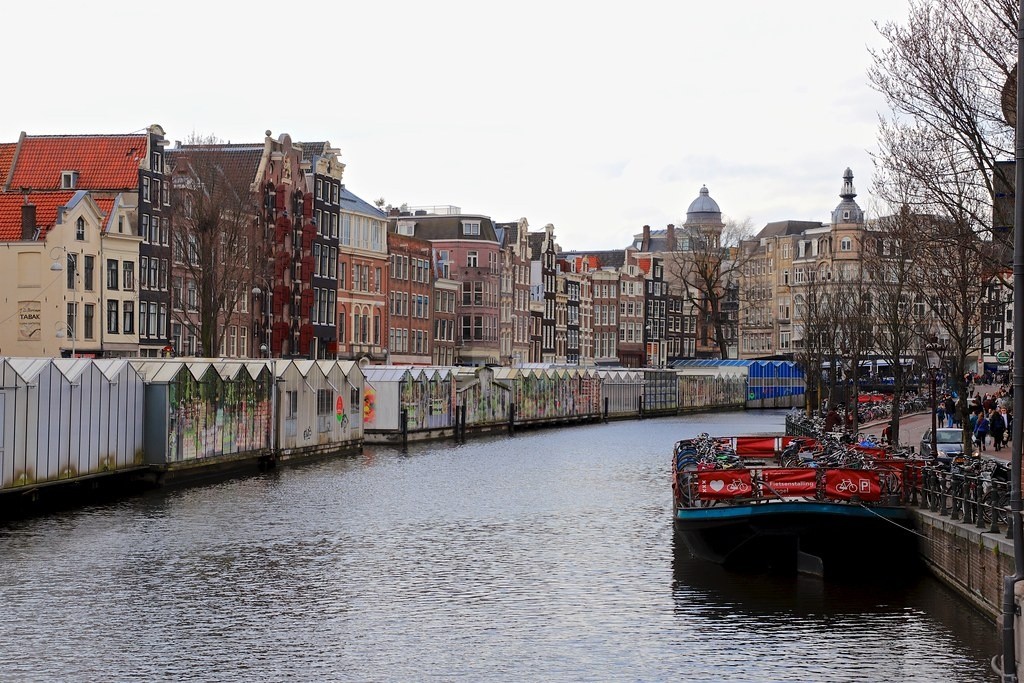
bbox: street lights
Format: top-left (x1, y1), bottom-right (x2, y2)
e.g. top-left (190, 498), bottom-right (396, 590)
top-left (923, 337), bottom-right (945, 464)
top-left (47, 246), bottom-right (76, 358)
top-left (250, 274), bottom-right (272, 359)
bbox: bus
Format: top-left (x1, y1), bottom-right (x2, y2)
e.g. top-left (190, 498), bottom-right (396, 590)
top-left (822, 358), bottom-right (923, 386)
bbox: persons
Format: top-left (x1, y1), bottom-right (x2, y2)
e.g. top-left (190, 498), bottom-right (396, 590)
top-left (647, 359), bottom-right (653, 368)
top-left (824, 363), bottom-right (1014, 452)
top-left (164, 349), bottom-right (202, 357)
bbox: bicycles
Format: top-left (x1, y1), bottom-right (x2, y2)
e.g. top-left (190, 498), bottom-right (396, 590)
top-left (673, 388), bottom-right (1012, 528)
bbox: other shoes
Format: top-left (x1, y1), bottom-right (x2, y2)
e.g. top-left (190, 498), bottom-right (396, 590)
top-left (995, 449), bottom-right (998, 451)
top-left (983, 445), bottom-right (986, 451)
top-left (1001, 445), bottom-right (1005, 448)
top-left (999, 446), bottom-right (1001, 450)
top-left (946, 426), bottom-right (953, 428)
top-left (980, 447), bottom-right (982, 452)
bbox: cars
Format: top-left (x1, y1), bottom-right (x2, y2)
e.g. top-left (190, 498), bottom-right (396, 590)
top-left (919, 427), bottom-right (981, 469)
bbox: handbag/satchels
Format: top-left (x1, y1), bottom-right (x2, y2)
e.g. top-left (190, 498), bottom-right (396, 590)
top-left (975, 429), bottom-right (978, 439)
top-left (881, 428), bottom-right (889, 443)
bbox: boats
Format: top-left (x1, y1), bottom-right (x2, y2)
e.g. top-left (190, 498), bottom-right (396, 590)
top-left (671, 433), bottom-right (922, 581)
